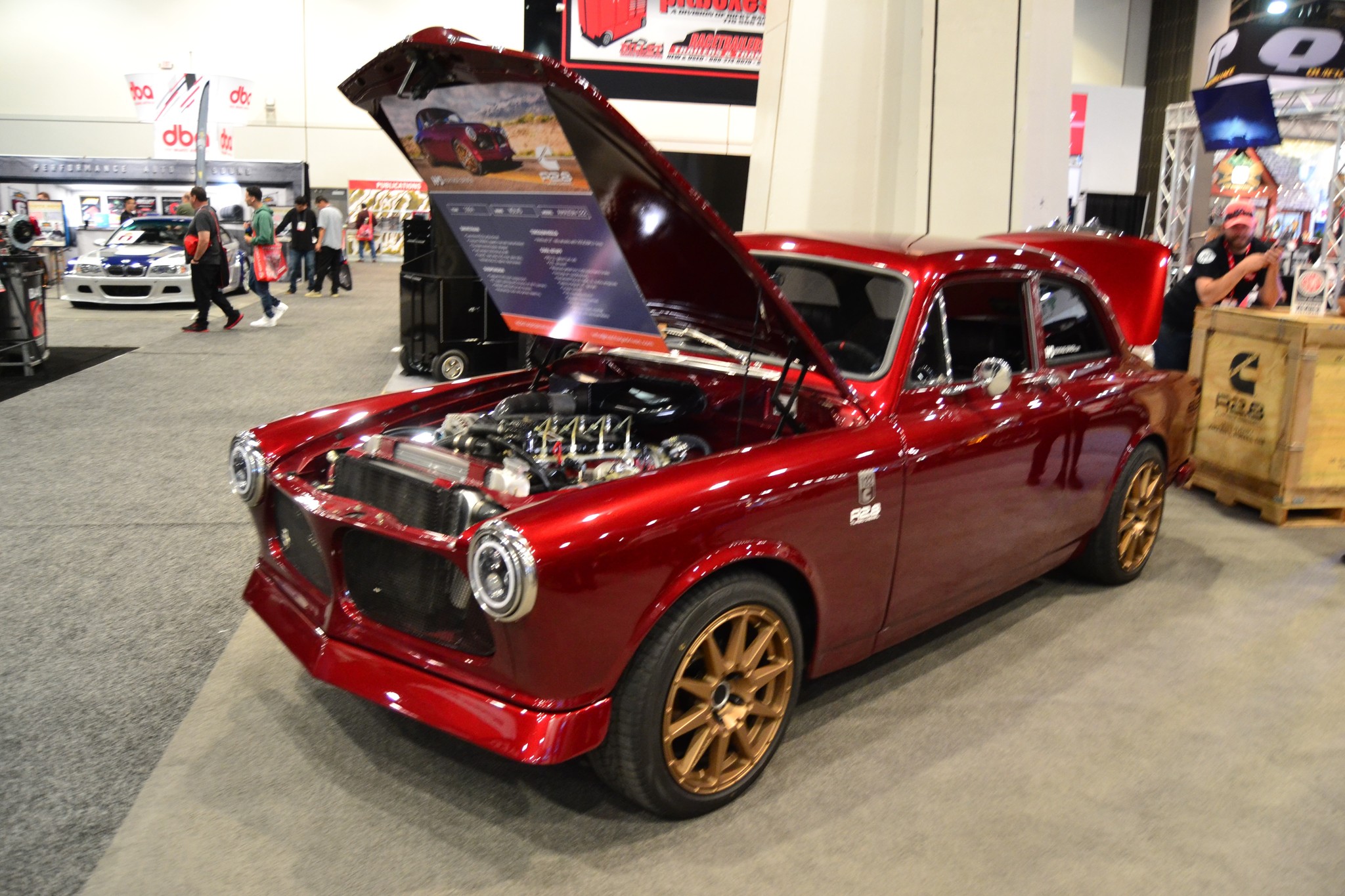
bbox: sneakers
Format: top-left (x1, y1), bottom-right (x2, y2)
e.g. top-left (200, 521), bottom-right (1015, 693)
top-left (183, 321), bottom-right (209, 332)
top-left (273, 303), bottom-right (289, 321)
top-left (223, 310), bottom-right (244, 329)
top-left (331, 291), bottom-right (340, 297)
top-left (306, 289), bottom-right (322, 297)
top-left (250, 316), bottom-right (277, 327)
top-left (286, 287), bottom-right (296, 294)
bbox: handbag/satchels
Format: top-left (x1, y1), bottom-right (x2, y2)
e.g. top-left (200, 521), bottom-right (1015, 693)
top-left (355, 224), bottom-right (375, 241)
top-left (216, 247), bottom-right (230, 288)
top-left (183, 236), bottom-right (211, 256)
top-left (253, 242), bottom-right (287, 282)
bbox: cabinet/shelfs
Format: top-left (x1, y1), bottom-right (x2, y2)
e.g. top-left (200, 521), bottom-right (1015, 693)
top-left (1184, 308), bottom-right (1345, 522)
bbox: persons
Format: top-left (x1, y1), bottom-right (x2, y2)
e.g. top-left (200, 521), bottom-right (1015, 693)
top-left (36, 191), bottom-right (54, 289)
top-left (1020, 404), bottom-right (1090, 491)
top-left (181, 186), bottom-right (244, 333)
top-left (243, 186), bottom-right (289, 328)
top-left (274, 195), bottom-right (319, 296)
top-left (355, 203), bottom-right (377, 263)
top-left (1152, 202), bottom-right (1286, 374)
top-left (120, 197), bottom-right (138, 225)
top-left (167, 191), bottom-right (195, 230)
top-left (303, 195), bottom-right (344, 298)
top-left (51, 222), bottom-right (64, 238)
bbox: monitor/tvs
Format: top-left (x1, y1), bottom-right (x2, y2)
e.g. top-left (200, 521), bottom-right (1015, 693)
top-left (1189, 77), bottom-right (1282, 152)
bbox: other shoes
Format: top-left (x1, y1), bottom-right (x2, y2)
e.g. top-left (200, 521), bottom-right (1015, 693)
top-left (1052, 472), bottom-right (1066, 489)
top-left (373, 260), bottom-right (378, 262)
top-left (356, 259), bottom-right (364, 262)
top-left (1069, 474), bottom-right (1083, 487)
top-left (1026, 471), bottom-right (1041, 485)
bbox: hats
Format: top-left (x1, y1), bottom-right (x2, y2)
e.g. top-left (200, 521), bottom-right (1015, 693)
top-left (1222, 203), bottom-right (1258, 229)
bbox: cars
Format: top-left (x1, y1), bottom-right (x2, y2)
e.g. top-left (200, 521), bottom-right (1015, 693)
top-left (415, 107), bottom-right (514, 175)
top-left (231, 27), bottom-right (1205, 818)
top-left (66, 215), bottom-right (251, 304)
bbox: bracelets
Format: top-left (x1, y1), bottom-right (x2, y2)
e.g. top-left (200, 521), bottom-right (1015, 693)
top-left (193, 258), bottom-right (200, 262)
